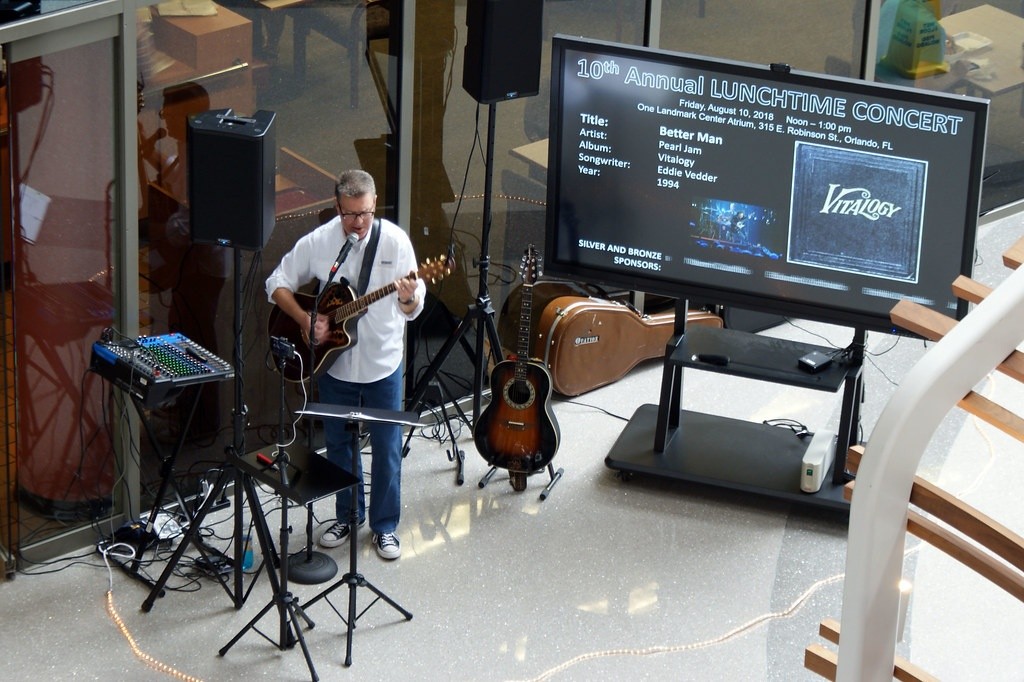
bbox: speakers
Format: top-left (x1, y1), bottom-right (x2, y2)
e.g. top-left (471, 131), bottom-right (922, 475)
top-left (462, 0), bottom-right (544, 105)
top-left (181, 107), bottom-right (277, 247)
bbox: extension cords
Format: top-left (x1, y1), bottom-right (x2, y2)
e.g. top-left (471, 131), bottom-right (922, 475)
top-left (206, 499), bottom-right (230, 515)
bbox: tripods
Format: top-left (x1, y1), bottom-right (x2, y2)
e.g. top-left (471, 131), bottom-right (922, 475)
top-left (142, 238), bottom-right (420, 682)
top-left (402, 102), bottom-right (506, 439)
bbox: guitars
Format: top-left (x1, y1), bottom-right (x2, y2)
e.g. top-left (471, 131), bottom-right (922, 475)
top-left (264, 250), bottom-right (462, 385)
top-left (470, 241), bottom-right (562, 476)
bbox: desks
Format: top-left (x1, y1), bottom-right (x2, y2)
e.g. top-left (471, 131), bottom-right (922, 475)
top-left (937, 3), bottom-right (1024, 99)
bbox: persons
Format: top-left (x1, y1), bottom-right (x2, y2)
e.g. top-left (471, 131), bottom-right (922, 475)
top-left (150, 82), bottom-right (226, 430)
top-left (265, 170), bottom-right (426, 559)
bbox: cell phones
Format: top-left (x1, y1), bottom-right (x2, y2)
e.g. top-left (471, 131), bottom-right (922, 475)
top-left (268, 335), bottom-right (296, 359)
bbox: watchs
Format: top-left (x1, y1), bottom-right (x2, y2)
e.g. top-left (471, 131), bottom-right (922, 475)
top-left (398, 295), bottom-right (415, 305)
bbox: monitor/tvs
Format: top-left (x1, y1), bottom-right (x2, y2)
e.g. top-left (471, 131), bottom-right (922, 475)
top-left (541, 32), bottom-right (989, 339)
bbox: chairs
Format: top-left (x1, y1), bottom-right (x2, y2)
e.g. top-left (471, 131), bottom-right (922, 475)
top-left (500, 67), bottom-right (553, 312)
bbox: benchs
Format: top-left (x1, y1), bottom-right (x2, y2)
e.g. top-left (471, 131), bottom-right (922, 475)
top-left (283, 0), bottom-right (390, 114)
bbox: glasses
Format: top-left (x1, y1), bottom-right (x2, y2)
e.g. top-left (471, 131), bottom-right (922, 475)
top-left (339, 205), bottom-right (374, 220)
top-left (158, 109), bottom-right (184, 119)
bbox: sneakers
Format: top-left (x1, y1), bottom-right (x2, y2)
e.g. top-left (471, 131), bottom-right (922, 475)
top-left (320, 517), bottom-right (366, 549)
top-left (373, 525), bottom-right (401, 559)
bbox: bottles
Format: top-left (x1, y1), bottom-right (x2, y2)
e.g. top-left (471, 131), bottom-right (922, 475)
top-left (242, 523), bottom-right (253, 570)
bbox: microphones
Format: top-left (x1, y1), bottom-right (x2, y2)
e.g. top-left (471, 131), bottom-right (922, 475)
top-left (328, 233), bottom-right (358, 276)
